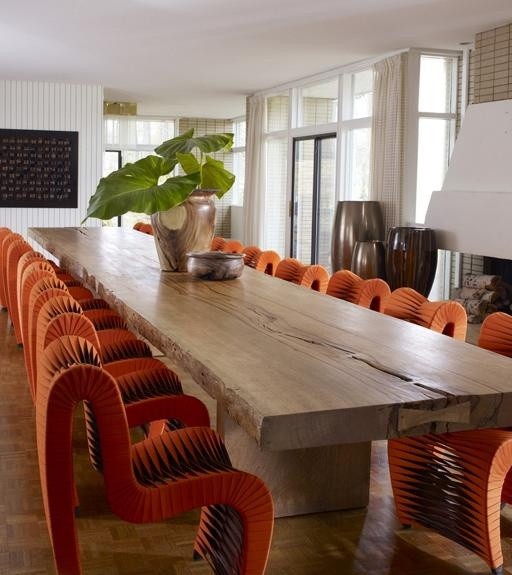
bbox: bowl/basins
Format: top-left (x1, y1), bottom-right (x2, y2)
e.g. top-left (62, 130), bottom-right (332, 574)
top-left (185, 251), bottom-right (246, 280)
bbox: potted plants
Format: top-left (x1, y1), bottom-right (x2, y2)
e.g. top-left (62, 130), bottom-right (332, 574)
top-left (79, 128), bottom-right (236, 273)
